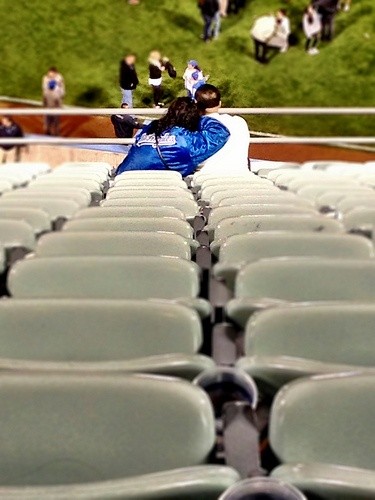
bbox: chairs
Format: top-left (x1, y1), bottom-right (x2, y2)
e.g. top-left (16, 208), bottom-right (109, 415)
top-left (0, 161), bottom-right (375, 500)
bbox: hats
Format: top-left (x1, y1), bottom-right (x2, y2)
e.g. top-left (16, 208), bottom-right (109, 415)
top-left (192, 72), bottom-right (198, 80)
top-left (187, 60), bottom-right (197, 67)
top-left (48, 80), bottom-right (56, 89)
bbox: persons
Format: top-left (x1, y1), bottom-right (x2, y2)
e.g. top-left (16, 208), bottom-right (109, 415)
top-left (42, 67), bottom-right (64, 136)
top-left (0, 115), bottom-right (23, 163)
top-left (277, 9), bottom-right (291, 52)
top-left (198, 0), bottom-right (228, 42)
top-left (252, 15), bottom-right (277, 63)
top-left (148, 51), bottom-right (170, 107)
top-left (312, 0), bottom-right (338, 42)
top-left (115, 96), bottom-right (230, 177)
top-left (195, 84), bottom-right (250, 170)
top-left (303, 5), bottom-right (321, 55)
top-left (183, 61), bottom-right (207, 100)
top-left (111, 103), bottom-right (147, 138)
top-left (119, 53), bottom-right (140, 117)
